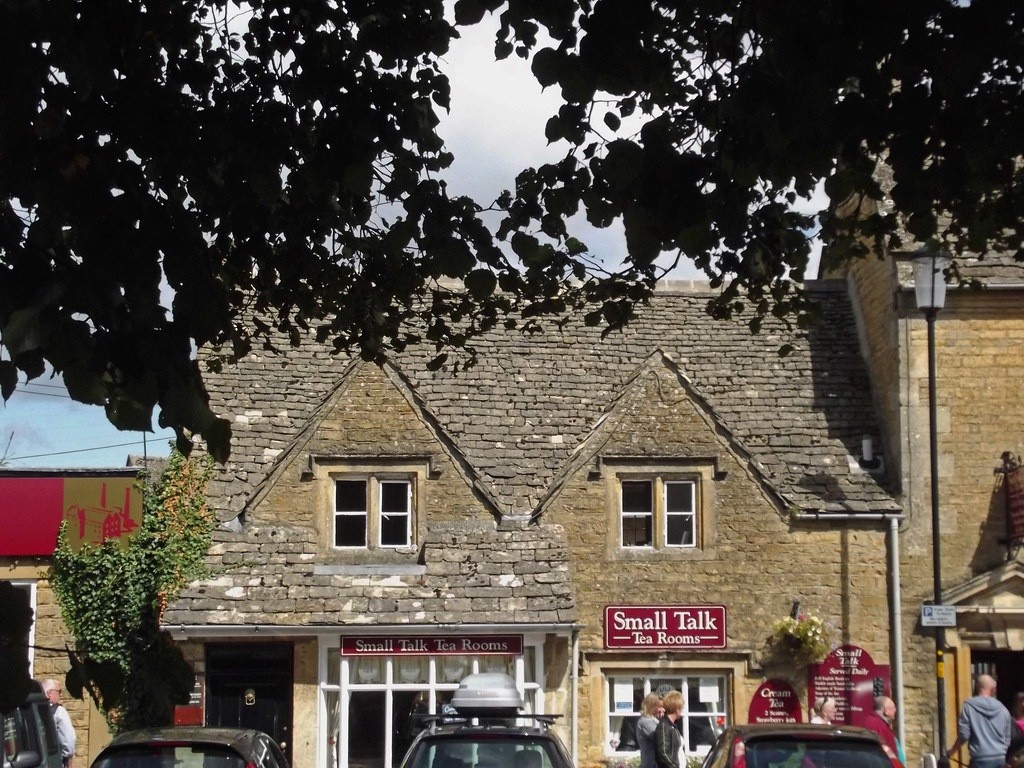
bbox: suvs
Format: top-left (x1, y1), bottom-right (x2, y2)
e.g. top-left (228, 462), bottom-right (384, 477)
top-left (1, 680), bottom-right (63, 768)
top-left (703, 724), bottom-right (907, 768)
top-left (399, 714), bottom-right (577, 768)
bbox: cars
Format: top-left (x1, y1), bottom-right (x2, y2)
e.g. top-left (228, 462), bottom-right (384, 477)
top-left (89, 727), bottom-right (290, 768)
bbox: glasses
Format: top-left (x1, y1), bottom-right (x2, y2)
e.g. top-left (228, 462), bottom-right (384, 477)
top-left (52, 688), bottom-right (62, 694)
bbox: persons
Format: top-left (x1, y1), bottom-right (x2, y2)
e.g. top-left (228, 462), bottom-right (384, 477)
top-left (810, 698), bottom-right (836, 725)
top-left (863, 696), bottom-right (906, 768)
top-left (41, 678), bottom-right (76, 768)
top-left (655, 691), bottom-right (687, 768)
top-left (636, 693), bottom-right (665, 768)
top-left (1005, 692), bottom-right (1024, 768)
top-left (946, 674), bottom-right (1011, 768)
